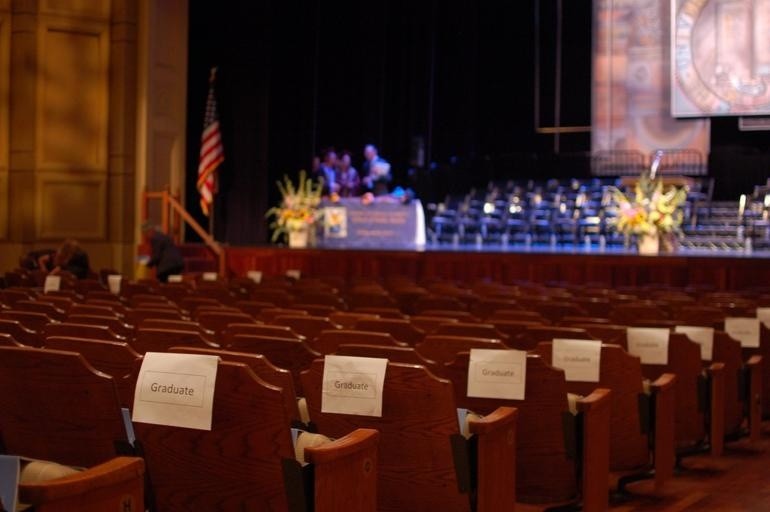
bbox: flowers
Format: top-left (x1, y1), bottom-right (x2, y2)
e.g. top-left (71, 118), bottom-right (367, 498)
top-left (600, 167), bottom-right (691, 236)
top-left (264, 169), bottom-right (325, 244)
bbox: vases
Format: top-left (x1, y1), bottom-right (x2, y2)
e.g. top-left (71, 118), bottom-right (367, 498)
top-left (635, 232), bottom-right (660, 258)
top-left (287, 231), bottom-right (310, 250)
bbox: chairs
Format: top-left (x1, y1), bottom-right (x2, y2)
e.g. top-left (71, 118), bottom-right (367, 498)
top-left (0, 268), bottom-right (770, 512)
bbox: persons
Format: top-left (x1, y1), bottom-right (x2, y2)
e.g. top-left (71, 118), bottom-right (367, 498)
top-left (141, 218), bottom-right (183, 283)
top-left (307, 143), bottom-right (392, 196)
top-left (54, 237), bottom-right (89, 279)
top-left (25, 249), bottom-right (62, 275)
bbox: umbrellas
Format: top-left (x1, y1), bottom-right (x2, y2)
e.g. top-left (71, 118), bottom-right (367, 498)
top-left (196, 77), bottom-right (226, 217)
